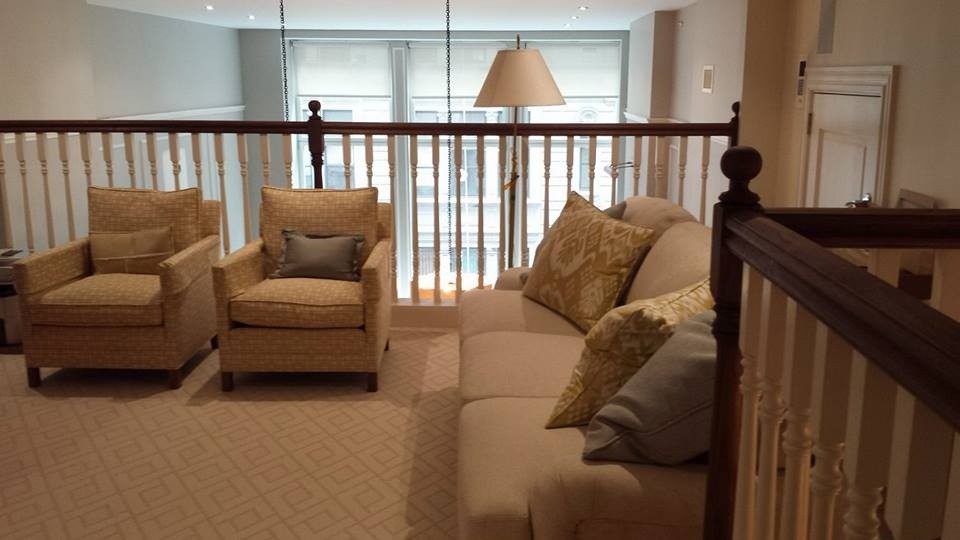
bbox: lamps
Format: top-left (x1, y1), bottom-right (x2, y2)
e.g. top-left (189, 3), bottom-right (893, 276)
top-left (473, 34), bottom-right (566, 268)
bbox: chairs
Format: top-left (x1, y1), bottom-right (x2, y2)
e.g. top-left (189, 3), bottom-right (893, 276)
top-left (14, 187), bottom-right (225, 390)
top-left (210, 187), bottom-right (396, 393)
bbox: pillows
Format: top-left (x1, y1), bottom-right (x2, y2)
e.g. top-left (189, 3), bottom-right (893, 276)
top-left (87, 226), bottom-right (175, 276)
top-left (581, 306), bottom-right (718, 462)
top-left (269, 228), bottom-right (366, 281)
top-left (523, 191), bottom-right (653, 324)
top-left (543, 277), bottom-right (716, 430)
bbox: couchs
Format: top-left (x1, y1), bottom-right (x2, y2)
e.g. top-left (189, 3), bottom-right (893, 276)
top-left (458, 195), bottom-right (712, 538)
